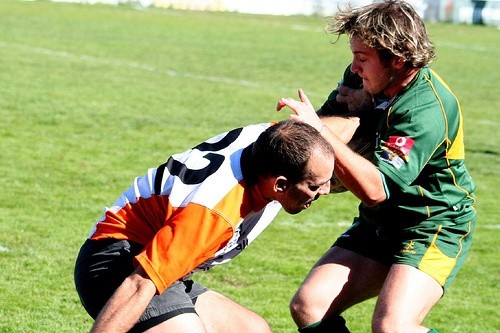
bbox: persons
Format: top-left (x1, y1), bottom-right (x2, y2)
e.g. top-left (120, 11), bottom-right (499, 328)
top-left (73, 102), bottom-right (374, 333)
top-left (275, 0), bottom-right (478, 332)
